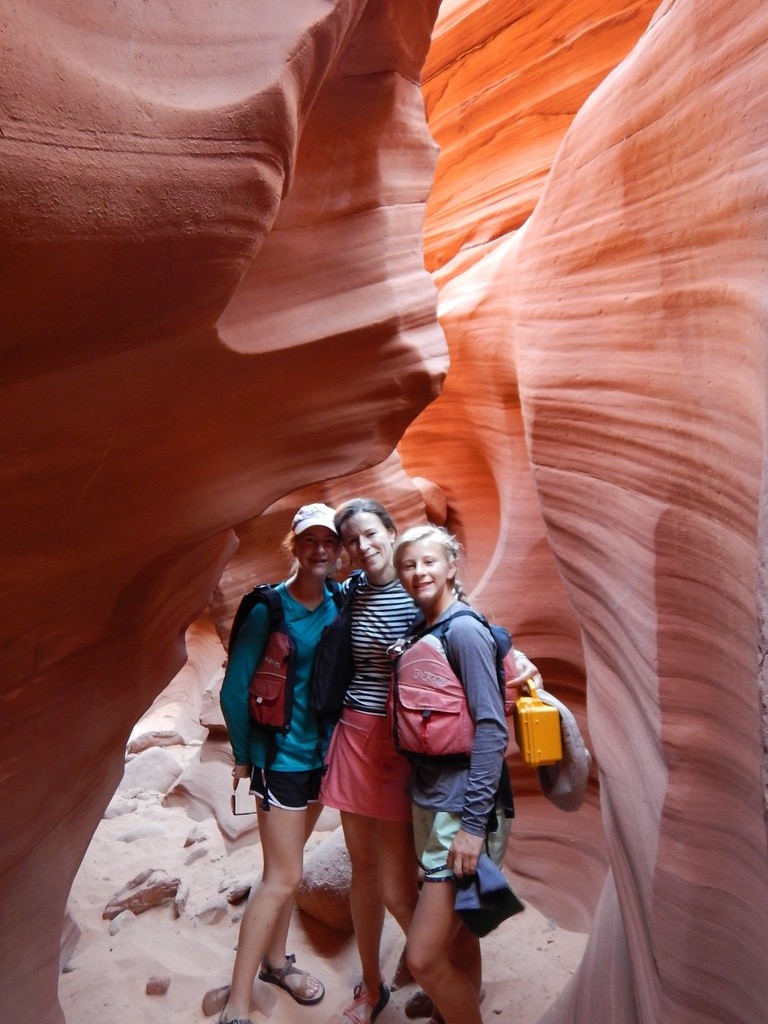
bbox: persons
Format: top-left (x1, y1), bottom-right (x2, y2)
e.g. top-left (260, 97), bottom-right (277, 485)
top-left (393, 525), bottom-right (515, 1024)
top-left (220, 502), bottom-right (335, 1024)
top-left (318, 499), bottom-right (543, 1024)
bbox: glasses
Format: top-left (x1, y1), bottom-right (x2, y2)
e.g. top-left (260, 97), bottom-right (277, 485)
top-left (231, 777), bottom-right (264, 816)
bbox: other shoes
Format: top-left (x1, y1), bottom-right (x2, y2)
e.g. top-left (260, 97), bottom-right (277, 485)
top-left (405, 990), bottom-right (434, 1019)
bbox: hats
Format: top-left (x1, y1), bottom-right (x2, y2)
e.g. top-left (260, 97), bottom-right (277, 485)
top-left (292, 503), bottom-right (338, 535)
top-left (533, 688), bottom-right (593, 812)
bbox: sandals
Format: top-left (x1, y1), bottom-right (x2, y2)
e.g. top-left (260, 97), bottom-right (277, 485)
top-left (259, 954), bottom-right (325, 1003)
top-left (219, 1002), bottom-right (251, 1024)
top-left (338, 973), bottom-right (390, 1024)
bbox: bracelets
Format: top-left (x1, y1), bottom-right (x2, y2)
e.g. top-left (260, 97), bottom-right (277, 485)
top-left (515, 654), bottom-right (527, 659)
